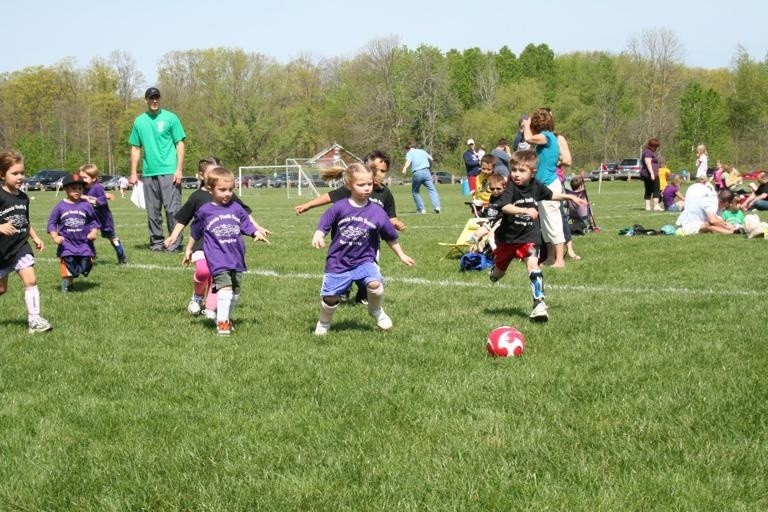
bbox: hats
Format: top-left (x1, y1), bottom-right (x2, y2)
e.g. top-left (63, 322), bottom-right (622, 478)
top-left (144, 87), bottom-right (160, 98)
top-left (466, 138), bottom-right (475, 146)
top-left (63, 174), bottom-right (88, 186)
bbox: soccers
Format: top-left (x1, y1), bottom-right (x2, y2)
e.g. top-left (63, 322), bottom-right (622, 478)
top-left (486, 326), bottom-right (525, 358)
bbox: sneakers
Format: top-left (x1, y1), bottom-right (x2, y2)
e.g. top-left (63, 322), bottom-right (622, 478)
top-left (368, 306), bottom-right (393, 330)
top-left (119, 254), bottom-right (128, 264)
top-left (216, 318), bottom-right (235, 337)
top-left (28, 313), bottom-right (53, 334)
top-left (205, 309), bottom-right (217, 321)
top-left (489, 265), bottom-right (506, 282)
top-left (313, 320), bottom-right (331, 336)
top-left (62, 278), bottom-right (74, 293)
top-left (355, 295), bottom-right (368, 305)
top-left (339, 292), bottom-right (349, 304)
top-left (188, 296), bottom-right (203, 316)
top-left (529, 300), bottom-right (549, 322)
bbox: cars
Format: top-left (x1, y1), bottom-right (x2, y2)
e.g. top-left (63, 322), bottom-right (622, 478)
top-left (431, 171), bottom-right (462, 184)
top-left (385, 175), bottom-right (401, 186)
top-left (705, 165), bottom-right (766, 179)
top-left (587, 157), bottom-right (642, 181)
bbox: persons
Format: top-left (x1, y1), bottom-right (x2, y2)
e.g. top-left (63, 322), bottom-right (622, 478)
top-left (311, 162), bottom-right (417, 336)
top-left (1, 149), bottom-right (53, 338)
top-left (462, 105), bottom-right (596, 324)
top-left (180, 164), bottom-right (271, 336)
top-left (77, 160), bottom-right (127, 264)
top-left (116, 172), bottom-right (129, 200)
top-left (47, 172), bottom-right (102, 295)
top-left (641, 134), bottom-right (768, 242)
top-left (402, 138), bottom-right (442, 216)
top-left (125, 84), bottom-right (186, 252)
top-left (295, 148), bottom-right (408, 309)
top-left (164, 155), bottom-right (274, 321)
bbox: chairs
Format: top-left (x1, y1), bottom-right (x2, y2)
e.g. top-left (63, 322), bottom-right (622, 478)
top-left (436, 216), bottom-right (496, 264)
